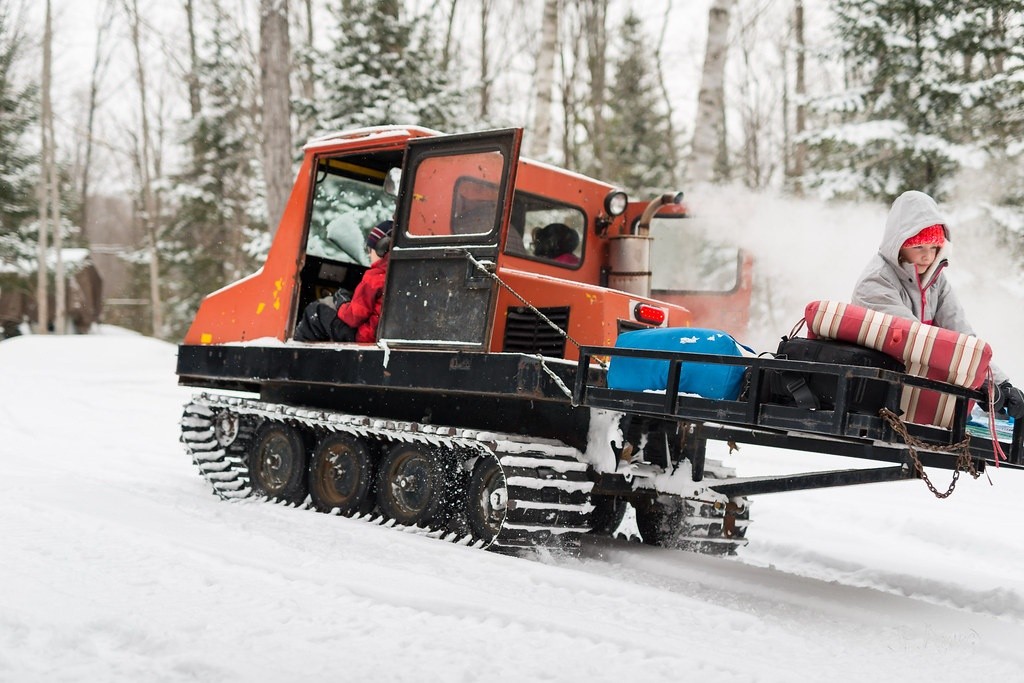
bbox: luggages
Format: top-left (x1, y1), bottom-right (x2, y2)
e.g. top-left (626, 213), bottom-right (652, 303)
top-left (606, 328), bottom-right (756, 400)
top-left (769, 335), bottom-right (905, 416)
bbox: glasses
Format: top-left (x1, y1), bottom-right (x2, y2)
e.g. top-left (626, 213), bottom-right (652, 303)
top-left (530, 243), bottom-right (538, 249)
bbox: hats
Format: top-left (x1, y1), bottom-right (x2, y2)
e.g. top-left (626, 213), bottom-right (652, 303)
top-left (901, 225), bottom-right (945, 250)
top-left (367, 220), bottom-right (393, 251)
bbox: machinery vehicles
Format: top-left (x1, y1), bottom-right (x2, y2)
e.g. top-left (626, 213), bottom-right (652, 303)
top-left (174, 124), bottom-right (1023, 556)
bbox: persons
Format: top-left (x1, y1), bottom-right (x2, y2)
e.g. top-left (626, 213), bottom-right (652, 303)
top-left (531, 222), bottom-right (582, 267)
top-left (293, 220), bottom-right (395, 344)
top-left (851, 189), bottom-right (1024, 422)
top-left (452, 176), bottom-right (529, 256)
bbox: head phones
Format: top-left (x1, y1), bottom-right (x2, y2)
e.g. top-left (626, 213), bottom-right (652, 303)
top-left (376, 236), bottom-right (393, 256)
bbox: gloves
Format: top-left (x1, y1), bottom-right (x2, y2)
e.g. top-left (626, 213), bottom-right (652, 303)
top-left (998, 383), bottom-right (1024, 419)
top-left (332, 289), bottom-right (351, 310)
top-left (976, 379), bottom-right (1004, 412)
